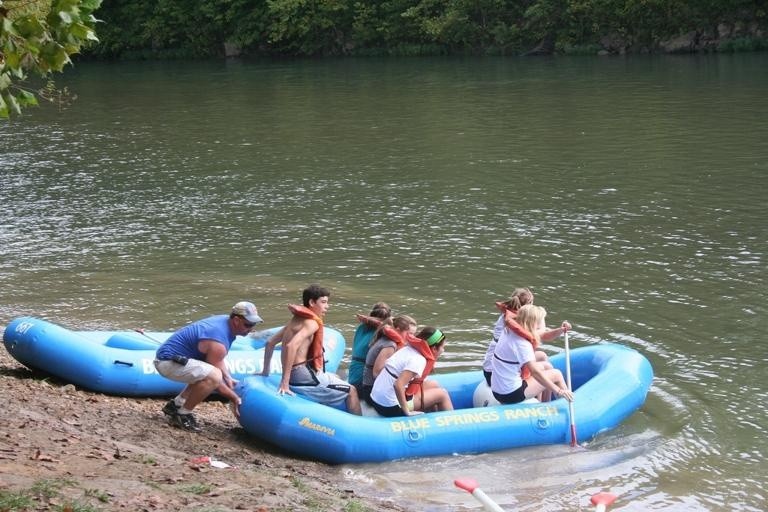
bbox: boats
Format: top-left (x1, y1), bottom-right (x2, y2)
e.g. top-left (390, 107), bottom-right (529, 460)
top-left (1, 310), bottom-right (351, 399)
top-left (222, 342), bottom-right (656, 469)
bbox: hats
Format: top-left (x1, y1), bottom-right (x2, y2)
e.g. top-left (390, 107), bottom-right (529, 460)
top-left (231, 302), bottom-right (264, 324)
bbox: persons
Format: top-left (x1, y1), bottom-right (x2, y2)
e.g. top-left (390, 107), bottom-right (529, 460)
top-left (369, 327), bottom-right (455, 416)
top-left (253, 284), bottom-right (362, 417)
top-left (362, 315), bottom-right (417, 407)
top-left (483, 287), bottom-right (554, 403)
top-left (153, 301), bottom-right (264, 432)
top-left (491, 304), bottom-right (575, 404)
top-left (348, 302), bottom-right (392, 399)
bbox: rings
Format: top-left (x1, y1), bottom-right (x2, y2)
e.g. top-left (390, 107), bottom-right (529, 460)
top-left (568, 392), bottom-right (571, 395)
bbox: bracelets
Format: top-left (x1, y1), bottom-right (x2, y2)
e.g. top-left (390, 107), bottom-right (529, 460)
top-left (558, 329), bottom-right (562, 333)
top-left (556, 387), bottom-right (564, 398)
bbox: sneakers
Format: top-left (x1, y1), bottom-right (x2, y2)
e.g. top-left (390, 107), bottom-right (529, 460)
top-left (162, 399), bottom-right (202, 433)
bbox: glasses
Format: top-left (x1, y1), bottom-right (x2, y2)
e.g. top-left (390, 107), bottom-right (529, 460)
top-left (239, 319), bottom-right (256, 327)
